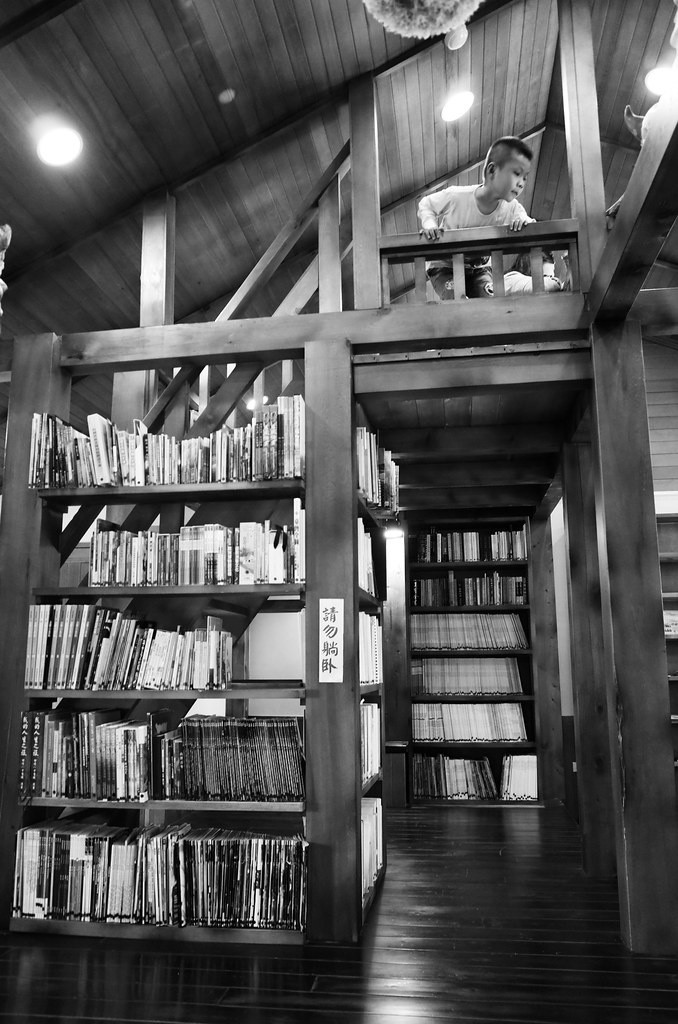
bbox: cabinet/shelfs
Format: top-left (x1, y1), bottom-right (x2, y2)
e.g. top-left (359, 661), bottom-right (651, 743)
top-left (387, 515), bottom-right (564, 808)
top-left (1, 292), bottom-right (386, 958)
top-left (565, 290), bottom-right (677, 957)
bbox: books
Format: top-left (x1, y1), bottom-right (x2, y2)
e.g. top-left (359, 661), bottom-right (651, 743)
top-left (88, 498), bottom-right (306, 587)
top-left (411, 613), bottom-right (528, 651)
top-left (410, 658), bottom-right (522, 695)
top-left (20, 707), bottom-right (305, 802)
top-left (409, 754), bottom-right (499, 801)
top-left (28, 394), bottom-right (306, 491)
top-left (412, 703), bottom-right (527, 743)
top-left (355, 425), bottom-right (400, 518)
top-left (358, 611), bottom-right (382, 903)
top-left (358, 517), bottom-right (376, 597)
top-left (499, 755), bottom-right (538, 801)
top-left (411, 570), bottom-right (528, 607)
top-left (13, 819), bottom-right (306, 932)
top-left (408, 523), bottom-right (528, 562)
top-left (25, 605), bottom-right (232, 691)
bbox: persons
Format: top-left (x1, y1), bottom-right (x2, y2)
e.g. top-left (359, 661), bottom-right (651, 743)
top-left (418, 136), bottom-right (563, 299)
